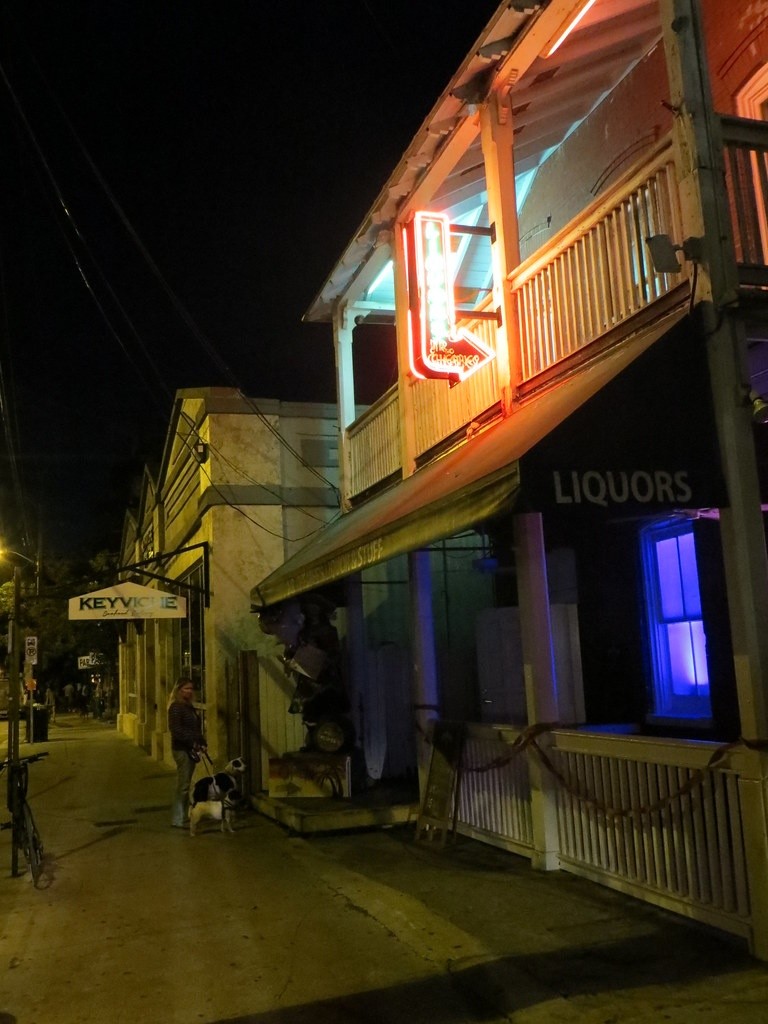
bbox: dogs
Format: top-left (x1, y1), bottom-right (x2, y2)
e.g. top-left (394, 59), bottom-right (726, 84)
top-left (188, 757), bottom-right (247, 837)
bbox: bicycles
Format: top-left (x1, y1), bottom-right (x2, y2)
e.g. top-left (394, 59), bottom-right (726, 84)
top-left (0, 751), bottom-right (51, 888)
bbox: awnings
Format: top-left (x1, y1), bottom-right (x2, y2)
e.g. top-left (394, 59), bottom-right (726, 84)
top-left (248, 304), bottom-right (699, 611)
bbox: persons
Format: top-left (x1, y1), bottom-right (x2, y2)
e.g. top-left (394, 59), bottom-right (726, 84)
top-left (36, 682), bottom-right (103, 721)
top-left (166, 675), bottom-right (208, 832)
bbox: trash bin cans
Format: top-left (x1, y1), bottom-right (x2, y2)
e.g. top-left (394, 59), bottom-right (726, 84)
top-left (26, 704), bottom-right (49, 743)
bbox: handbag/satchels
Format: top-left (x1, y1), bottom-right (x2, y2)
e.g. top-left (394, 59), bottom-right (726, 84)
top-left (188, 751), bottom-right (200, 762)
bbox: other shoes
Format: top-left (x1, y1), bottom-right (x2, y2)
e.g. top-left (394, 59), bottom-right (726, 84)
top-left (171, 821), bottom-right (190, 829)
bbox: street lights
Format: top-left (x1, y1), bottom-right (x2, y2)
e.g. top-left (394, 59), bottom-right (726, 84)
top-left (0, 529), bottom-right (42, 744)
